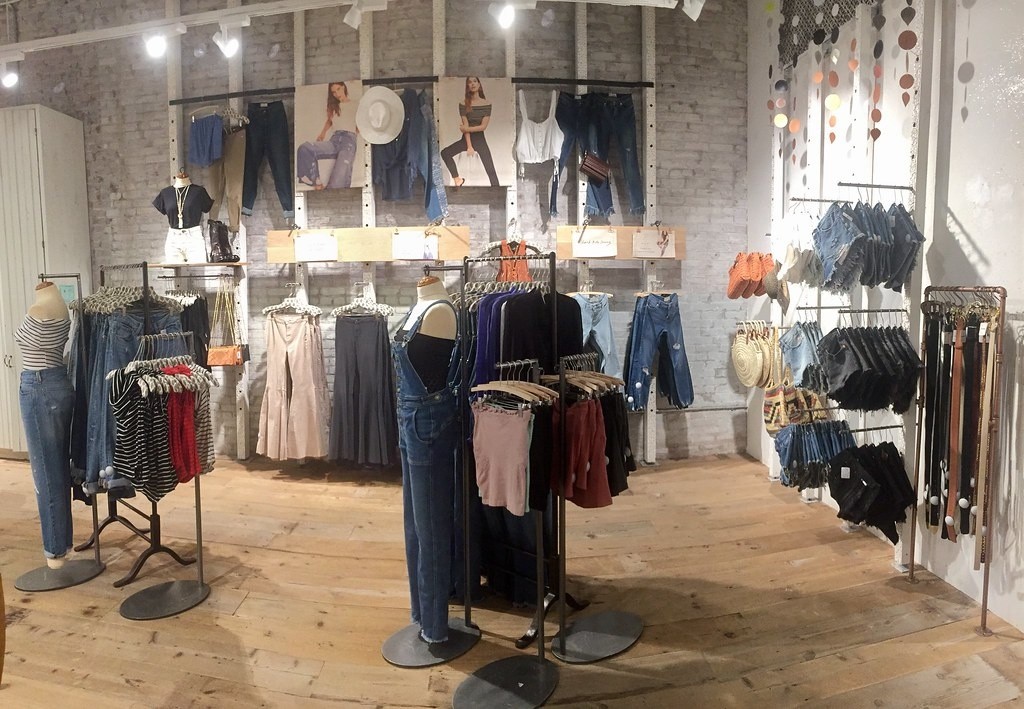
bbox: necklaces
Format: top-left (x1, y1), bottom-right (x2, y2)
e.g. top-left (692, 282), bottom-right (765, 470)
top-left (173, 182), bottom-right (192, 228)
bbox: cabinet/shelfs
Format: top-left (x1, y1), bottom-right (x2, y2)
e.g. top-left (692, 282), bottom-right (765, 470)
top-left (0, 104), bottom-right (93, 460)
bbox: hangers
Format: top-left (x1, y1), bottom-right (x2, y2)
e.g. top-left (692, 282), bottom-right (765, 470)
top-left (565, 280), bottom-right (614, 298)
top-left (262, 283), bottom-right (323, 317)
top-left (105, 332), bottom-right (220, 398)
top-left (220, 94), bottom-right (250, 126)
top-left (331, 279), bottom-right (395, 315)
top-left (478, 218), bottom-right (541, 258)
top-left (69, 264), bottom-right (203, 313)
top-left (470, 359), bottom-right (559, 402)
top-left (540, 353), bottom-right (625, 394)
top-left (633, 280), bottom-right (682, 297)
top-left (449, 254), bottom-right (550, 312)
top-left (189, 96), bottom-right (221, 114)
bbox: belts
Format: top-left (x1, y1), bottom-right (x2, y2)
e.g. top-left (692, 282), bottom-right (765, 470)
top-left (920, 301), bottom-right (999, 571)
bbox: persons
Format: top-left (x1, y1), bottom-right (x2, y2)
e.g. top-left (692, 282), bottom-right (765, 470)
top-left (296, 81), bottom-right (360, 189)
top-left (13, 282), bottom-right (75, 571)
top-left (440, 76), bottom-right (500, 188)
top-left (390, 274), bottom-right (463, 644)
top-left (153, 166), bottom-right (216, 265)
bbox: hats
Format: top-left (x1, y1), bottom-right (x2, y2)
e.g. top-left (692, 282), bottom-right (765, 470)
top-left (355, 86), bottom-right (405, 144)
top-left (761, 259), bottom-right (790, 316)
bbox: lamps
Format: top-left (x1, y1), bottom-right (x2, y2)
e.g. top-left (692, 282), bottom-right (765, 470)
top-left (141, 24), bottom-right (186, 58)
top-left (212, 14), bottom-right (250, 58)
top-left (0, 50), bottom-right (25, 87)
top-left (487, 0), bottom-right (537, 29)
top-left (343, 0), bottom-right (388, 30)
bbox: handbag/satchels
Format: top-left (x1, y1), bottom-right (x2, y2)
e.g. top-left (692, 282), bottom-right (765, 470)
top-left (207, 344), bottom-right (251, 367)
top-left (727, 252), bottom-right (775, 299)
top-left (579, 153), bottom-right (610, 183)
top-left (731, 320), bottom-right (827, 433)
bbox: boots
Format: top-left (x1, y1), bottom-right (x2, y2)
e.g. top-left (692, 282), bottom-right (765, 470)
top-left (219, 225), bottom-right (240, 262)
top-left (208, 219), bottom-right (229, 262)
